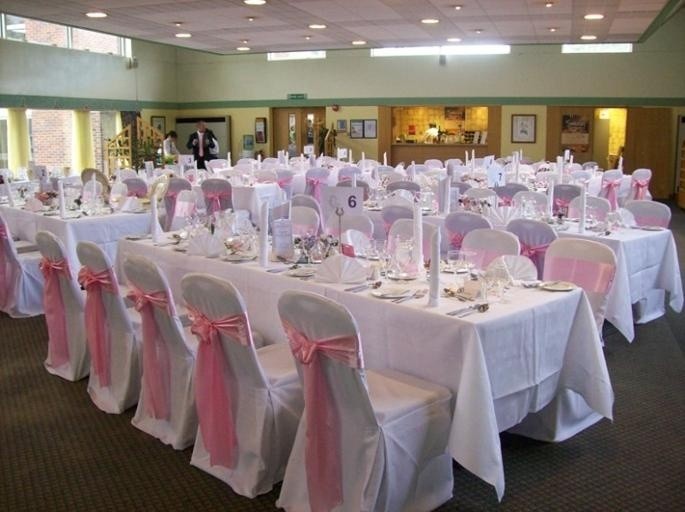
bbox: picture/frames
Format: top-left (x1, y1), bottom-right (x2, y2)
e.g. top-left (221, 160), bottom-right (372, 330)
top-left (511, 113), bottom-right (536, 143)
top-left (364, 119), bottom-right (377, 139)
top-left (150, 115), bottom-right (166, 149)
top-left (336, 120), bottom-right (347, 133)
top-left (350, 119), bottom-right (364, 139)
top-left (243, 134), bottom-right (254, 151)
top-left (255, 117), bottom-right (266, 144)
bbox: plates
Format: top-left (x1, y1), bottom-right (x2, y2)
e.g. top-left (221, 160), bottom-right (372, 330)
top-left (157, 230), bottom-right (579, 300)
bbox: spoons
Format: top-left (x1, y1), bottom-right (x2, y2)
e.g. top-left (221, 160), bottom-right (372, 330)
top-left (458, 304), bottom-right (489, 317)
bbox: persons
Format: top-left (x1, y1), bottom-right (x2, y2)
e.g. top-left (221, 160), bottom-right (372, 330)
top-left (205, 128), bottom-right (219, 160)
top-left (160, 130), bottom-right (179, 168)
top-left (187, 121), bottom-right (214, 169)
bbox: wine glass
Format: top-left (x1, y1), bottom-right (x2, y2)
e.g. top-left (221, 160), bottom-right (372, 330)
top-left (182, 208), bottom-right (515, 307)
top-left (95, 195), bottom-right (106, 217)
top-left (367, 184), bottom-right (626, 236)
